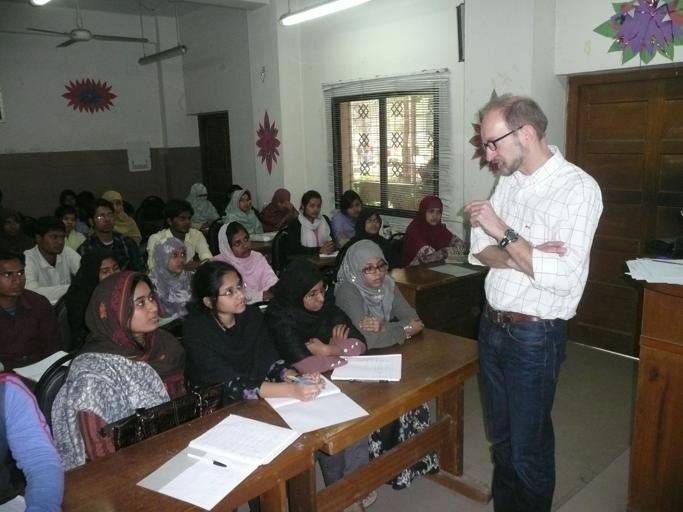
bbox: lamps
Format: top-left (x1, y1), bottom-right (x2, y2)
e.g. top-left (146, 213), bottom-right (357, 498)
top-left (276, 0), bottom-right (371, 30)
top-left (127, 1), bottom-right (188, 67)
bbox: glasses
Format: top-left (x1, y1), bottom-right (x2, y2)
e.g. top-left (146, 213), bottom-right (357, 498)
top-left (363, 263), bottom-right (387, 274)
top-left (481, 123), bottom-right (526, 152)
top-left (218, 281), bottom-right (248, 296)
top-left (305, 284), bottom-right (328, 299)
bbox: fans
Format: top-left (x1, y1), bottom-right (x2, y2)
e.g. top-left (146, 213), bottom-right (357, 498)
top-left (0, 1), bottom-right (151, 51)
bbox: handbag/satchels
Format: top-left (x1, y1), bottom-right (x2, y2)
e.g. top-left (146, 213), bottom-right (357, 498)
top-left (113, 381), bottom-right (226, 449)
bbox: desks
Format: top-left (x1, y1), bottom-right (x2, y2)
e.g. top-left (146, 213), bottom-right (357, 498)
top-left (0, 184), bottom-right (505, 512)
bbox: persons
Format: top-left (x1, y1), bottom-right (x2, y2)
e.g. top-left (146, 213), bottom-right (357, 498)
top-left (266, 259), bottom-right (377, 511)
top-left (463, 93), bottom-right (605, 512)
top-left (1, 191), bottom-right (281, 511)
top-left (177, 260), bottom-right (325, 510)
top-left (335, 239), bottom-right (441, 488)
top-left (185, 182), bottom-right (468, 299)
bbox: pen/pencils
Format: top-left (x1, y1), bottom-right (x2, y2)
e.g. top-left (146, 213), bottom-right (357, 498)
top-left (288, 376), bottom-right (311, 384)
top-left (187, 454), bottom-right (227, 468)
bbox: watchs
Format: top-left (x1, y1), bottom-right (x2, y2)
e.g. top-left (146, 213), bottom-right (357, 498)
top-left (498, 227), bottom-right (519, 247)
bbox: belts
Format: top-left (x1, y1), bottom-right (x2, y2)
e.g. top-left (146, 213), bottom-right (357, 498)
top-left (485, 302), bottom-right (543, 324)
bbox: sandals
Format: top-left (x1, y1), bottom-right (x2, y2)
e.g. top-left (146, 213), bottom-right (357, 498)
top-left (346, 490), bottom-right (377, 511)
